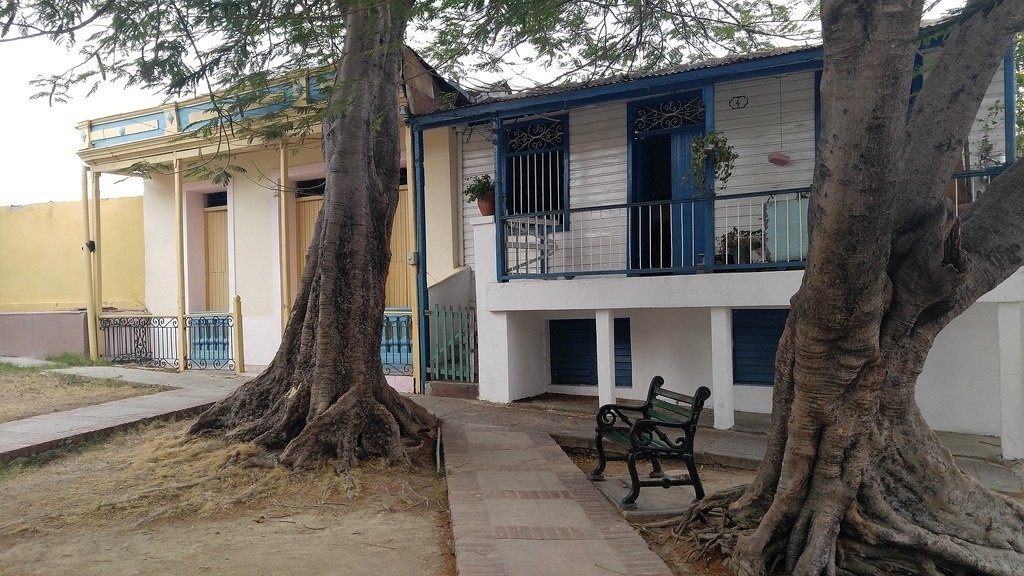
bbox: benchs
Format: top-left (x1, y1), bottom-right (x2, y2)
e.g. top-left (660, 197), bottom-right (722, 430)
top-left (588, 375), bottom-right (711, 511)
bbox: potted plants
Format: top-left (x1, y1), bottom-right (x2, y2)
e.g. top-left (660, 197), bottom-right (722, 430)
top-left (677, 129), bottom-right (739, 200)
top-left (462, 174), bottom-right (496, 216)
top-left (716, 228), bottom-right (770, 263)
